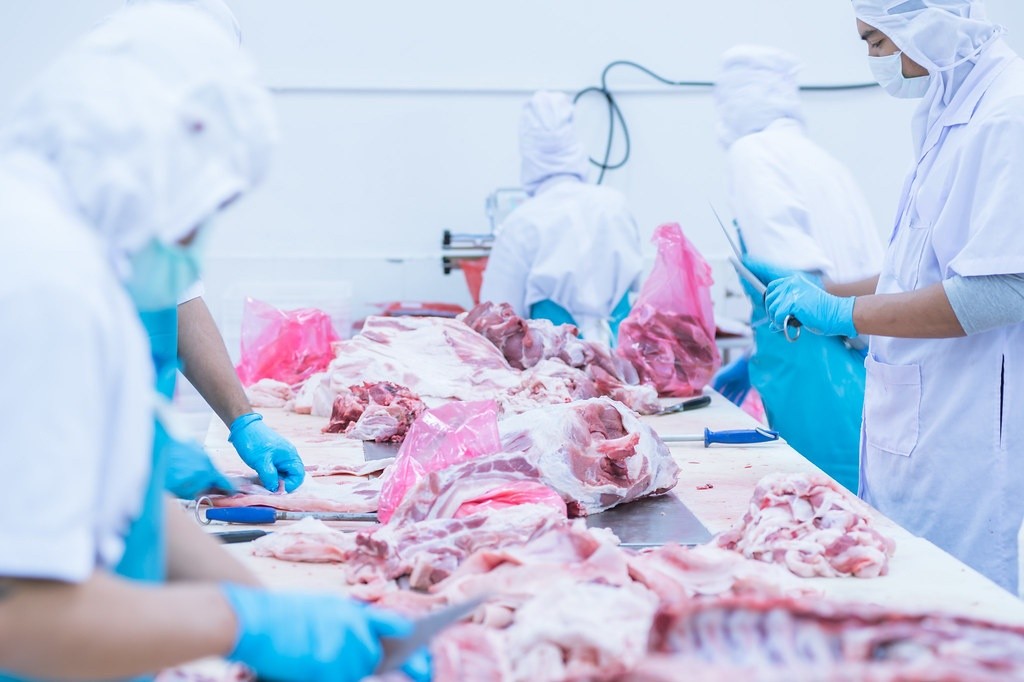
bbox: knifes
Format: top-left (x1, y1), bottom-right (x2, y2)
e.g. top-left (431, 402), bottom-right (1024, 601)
top-left (729, 257), bottom-right (803, 327)
top-left (208, 530), bottom-right (275, 544)
top-left (247, 589), bottom-right (488, 682)
top-left (706, 197), bottom-right (742, 271)
top-left (657, 396), bottom-right (712, 417)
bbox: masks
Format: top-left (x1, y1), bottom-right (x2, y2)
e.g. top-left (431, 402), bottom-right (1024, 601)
top-left (867, 51), bottom-right (930, 98)
top-left (125, 237), bottom-right (199, 313)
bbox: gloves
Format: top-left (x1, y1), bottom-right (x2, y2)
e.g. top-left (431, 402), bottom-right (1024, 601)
top-left (713, 356), bottom-right (752, 407)
top-left (221, 583), bottom-right (433, 682)
top-left (164, 438), bottom-right (235, 500)
top-left (735, 255), bottom-right (824, 315)
top-left (765, 276), bottom-right (859, 339)
top-left (228, 413), bottom-right (305, 493)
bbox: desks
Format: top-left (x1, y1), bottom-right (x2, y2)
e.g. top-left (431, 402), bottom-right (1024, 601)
top-left (156, 386), bottom-right (1023, 682)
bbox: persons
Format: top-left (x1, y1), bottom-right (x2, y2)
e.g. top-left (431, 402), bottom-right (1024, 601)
top-left (0, 2), bottom-right (434, 681)
top-left (477, 0), bottom-right (1024, 598)
